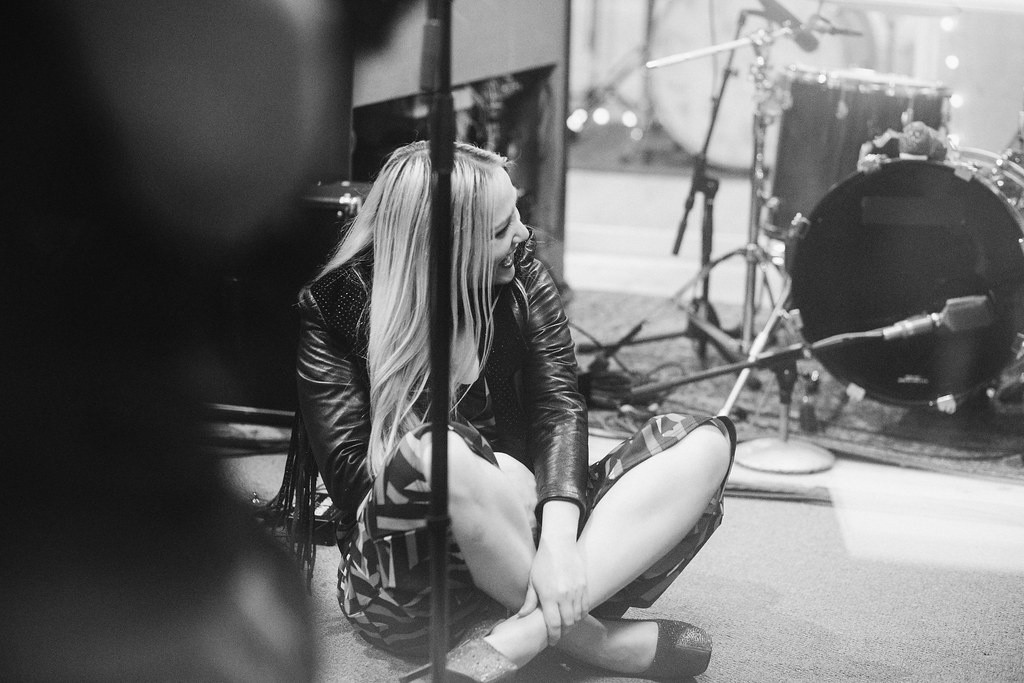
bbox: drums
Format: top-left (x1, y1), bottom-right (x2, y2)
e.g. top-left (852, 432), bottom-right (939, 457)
top-left (765, 60), bottom-right (949, 242)
top-left (781, 143), bottom-right (1024, 409)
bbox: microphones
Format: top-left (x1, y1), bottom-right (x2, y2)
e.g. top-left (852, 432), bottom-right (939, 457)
top-left (881, 296), bottom-right (994, 341)
top-left (760, 1), bottom-right (818, 52)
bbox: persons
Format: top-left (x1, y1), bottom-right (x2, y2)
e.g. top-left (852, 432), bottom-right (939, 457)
top-left (292, 136), bottom-right (738, 683)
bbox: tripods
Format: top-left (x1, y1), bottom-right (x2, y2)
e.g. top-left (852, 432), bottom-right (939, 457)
top-left (573, 1), bottom-right (862, 380)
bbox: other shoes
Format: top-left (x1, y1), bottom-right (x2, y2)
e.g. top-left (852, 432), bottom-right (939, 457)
top-left (554, 613), bottom-right (711, 678)
top-left (443, 618), bottom-right (518, 683)
